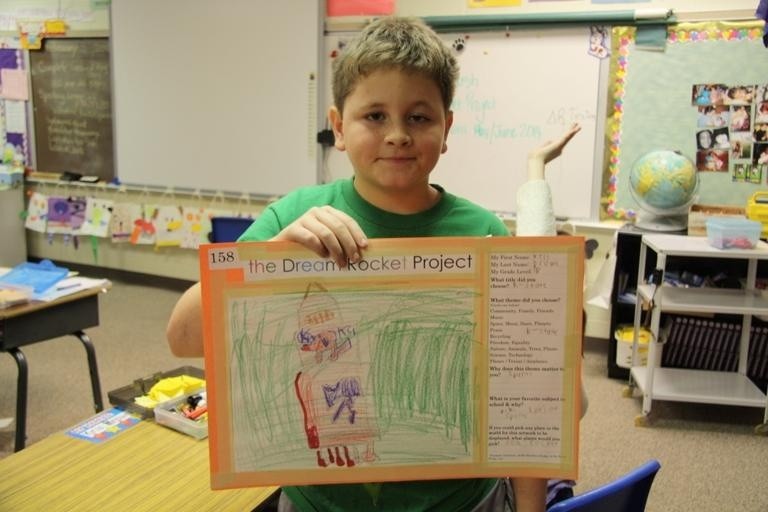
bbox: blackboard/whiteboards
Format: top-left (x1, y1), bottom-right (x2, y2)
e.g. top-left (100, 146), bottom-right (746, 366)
top-left (24, 23), bottom-right (612, 225)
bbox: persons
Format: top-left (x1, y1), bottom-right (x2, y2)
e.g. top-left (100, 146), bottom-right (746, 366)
top-left (696, 84), bottom-right (768, 177)
top-left (167, 14), bottom-right (551, 512)
top-left (514, 121), bottom-right (589, 423)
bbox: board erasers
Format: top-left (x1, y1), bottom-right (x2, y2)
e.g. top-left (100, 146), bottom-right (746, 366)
top-left (80, 175), bottom-right (99, 183)
top-left (60, 171), bottom-right (81, 182)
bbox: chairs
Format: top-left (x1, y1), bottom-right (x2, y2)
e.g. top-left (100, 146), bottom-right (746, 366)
top-left (544, 461), bottom-right (665, 512)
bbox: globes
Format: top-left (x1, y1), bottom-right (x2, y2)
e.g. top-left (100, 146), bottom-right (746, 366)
top-left (630, 144), bottom-right (700, 232)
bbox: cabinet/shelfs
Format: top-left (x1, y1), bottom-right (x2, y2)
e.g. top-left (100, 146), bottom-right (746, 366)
top-left (605, 222), bottom-right (767, 436)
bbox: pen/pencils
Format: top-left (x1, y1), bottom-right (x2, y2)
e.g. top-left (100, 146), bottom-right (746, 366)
top-left (57, 283), bottom-right (81, 291)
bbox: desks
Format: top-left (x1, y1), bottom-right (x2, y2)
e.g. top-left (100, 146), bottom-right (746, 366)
top-left (0, 267), bottom-right (110, 451)
top-left (0, 407), bottom-right (282, 512)
